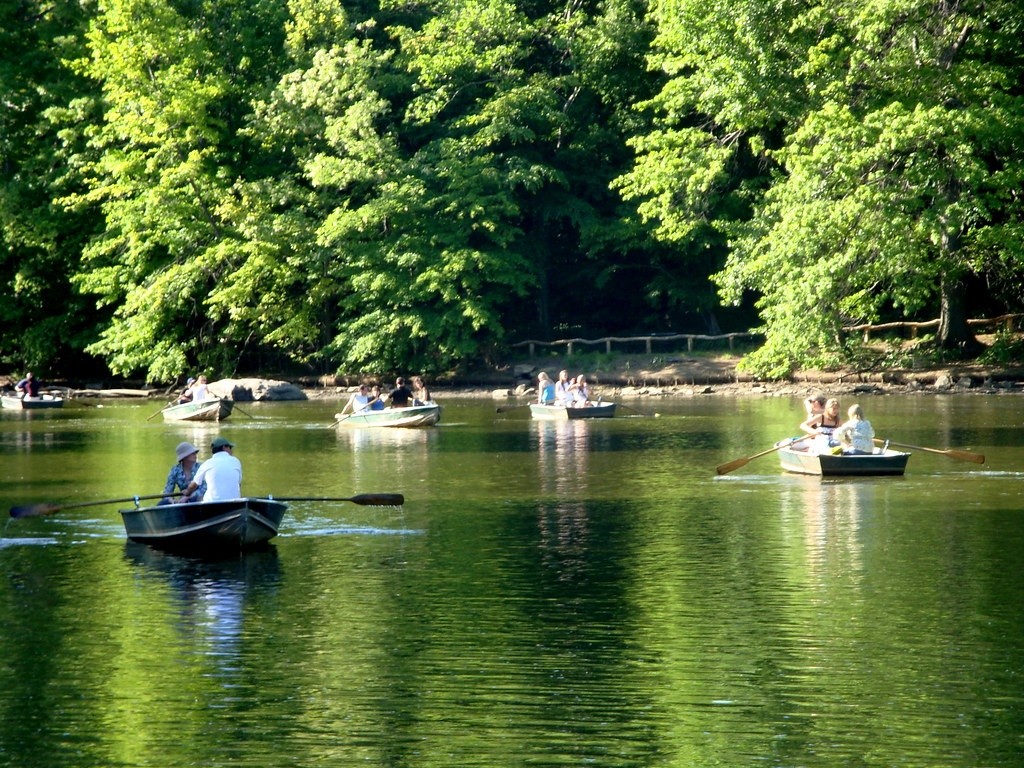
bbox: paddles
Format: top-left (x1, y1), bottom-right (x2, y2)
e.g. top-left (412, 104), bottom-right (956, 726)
top-left (208, 390), bottom-right (254, 420)
top-left (495, 403), bottom-right (534, 414)
top-left (9, 490), bottom-right (187, 519)
top-left (871, 436), bottom-right (987, 464)
top-left (38, 392), bottom-right (95, 407)
top-left (716, 430), bottom-right (821, 476)
top-left (582, 386), bottom-right (644, 415)
top-left (327, 396), bottom-right (382, 429)
top-left (251, 492), bottom-right (406, 507)
top-left (146, 394), bottom-right (186, 422)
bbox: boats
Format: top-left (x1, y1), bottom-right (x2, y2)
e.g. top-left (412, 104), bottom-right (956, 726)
top-left (116, 497), bottom-right (290, 561)
top-left (334, 400), bottom-right (442, 427)
top-left (160, 397), bottom-right (235, 423)
top-left (529, 400), bottom-right (617, 421)
top-left (772, 437), bottom-right (913, 476)
top-left (0, 384), bottom-right (65, 409)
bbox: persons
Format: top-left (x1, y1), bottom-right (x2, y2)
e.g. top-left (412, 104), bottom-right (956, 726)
top-left (800, 394), bottom-right (875, 455)
top-left (379, 377), bottom-right (413, 409)
top-left (368, 386), bottom-right (384, 410)
top-left (179, 375), bottom-right (209, 404)
top-left (156, 442), bottom-right (207, 506)
top-left (15, 373), bottom-right (40, 401)
top-left (182, 437), bottom-right (243, 501)
top-left (341, 383), bottom-right (369, 413)
top-left (537, 370), bottom-right (594, 407)
top-left (412, 378), bottom-right (431, 406)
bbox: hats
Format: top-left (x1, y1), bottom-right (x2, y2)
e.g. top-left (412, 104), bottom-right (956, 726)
top-left (176, 442), bottom-right (199, 462)
top-left (187, 377), bottom-right (195, 386)
top-left (211, 437), bottom-right (236, 449)
